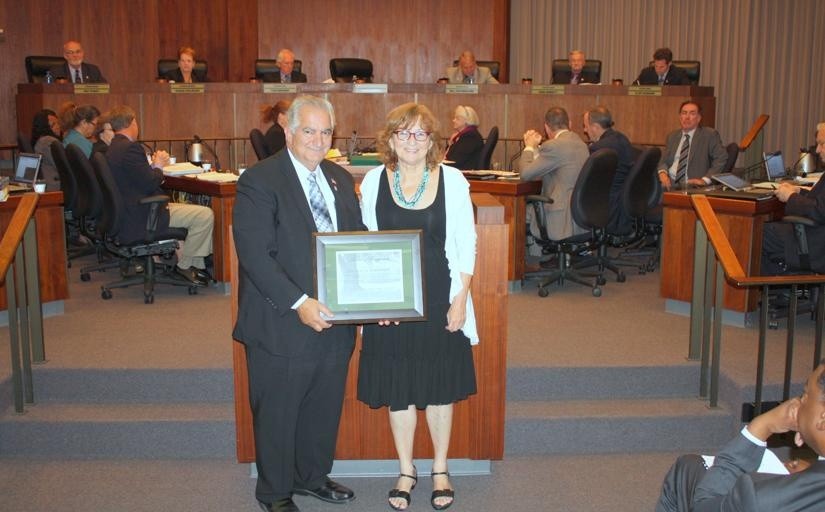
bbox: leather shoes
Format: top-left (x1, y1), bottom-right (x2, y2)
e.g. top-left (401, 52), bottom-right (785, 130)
top-left (294, 477), bottom-right (356, 503)
top-left (171, 264), bottom-right (208, 288)
top-left (256, 495), bottom-right (300, 512)
top-left (539, 257), bottom-right (574, 269)
top-left (190, 266), bottom-right (216, 286)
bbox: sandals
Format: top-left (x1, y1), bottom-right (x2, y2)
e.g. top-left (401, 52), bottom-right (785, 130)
top-left (430, 469), bottom-right (454, 510)
top-left (388, 465), bottom-right (418, 510)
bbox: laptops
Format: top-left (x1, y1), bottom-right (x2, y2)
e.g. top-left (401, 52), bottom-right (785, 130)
top-left (762, 150), bottom-right (804, 182)
top-left (711, 170), bottom-right (777, 196)
top-left (6, 151), bottom-right (44, 192)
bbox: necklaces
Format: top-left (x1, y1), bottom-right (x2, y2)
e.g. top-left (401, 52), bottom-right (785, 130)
top-left (390, 164), bottom-right (432, 209)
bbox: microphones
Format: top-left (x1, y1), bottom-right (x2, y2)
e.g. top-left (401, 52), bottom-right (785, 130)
top-left (792, 145), bottom-right (819, 173)
top-left (193, 134), bottom-right (221, 173)
top-left (737, 150), bottom-right (781, 179)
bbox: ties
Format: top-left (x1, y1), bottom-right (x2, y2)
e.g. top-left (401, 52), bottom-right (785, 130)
top-left (675, 134), bottom-right (691, 189)
top-left (74, 69), bottom-right (81, 83)
top-left (571, 74), bottom-right (578, 84)
top-left (307, 173), bottom-right (336, 232)
top-left (284, 75), bottom-right (289, 83)
top-left (659, 76), bottom-right (664, 85)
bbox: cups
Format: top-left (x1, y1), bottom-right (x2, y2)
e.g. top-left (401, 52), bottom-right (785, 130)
top-left (238, 163), bottom-right (249, 177)
top-left (521, 77), bottom-right (532, 85)
top-left (249, 77), bottom-right (265, 83)
top-left (167, 155), bottom-right (177, 164)
top-left (438, 77), bottom-right (449, 84)
top-left (55, 75), bottom-right (69, 83)
top-left (612, 78), bottom-right (624, 86)
top-left (155, 77), bottom-right (168, 83)
top-left (33, 179), bottom-right (46, 193)
top-left (201, 160), bottom-right (212, 172)
top-left (357, 77), bottom-right (369, 84)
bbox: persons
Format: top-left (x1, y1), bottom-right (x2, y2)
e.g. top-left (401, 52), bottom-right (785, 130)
top-left (29, 94), bottom-right (217, 298)
top-left (257, 48), bottom-right (308, 84)
top-left (753, 122), bottom-right (825, 309)
top-left (630, 46), bottom-right (695, 86)
top-left (228, 91), bottom-right (400, 510)
top-left (152, 46), bottom-right (214, 84)
top-left (579, 105), bottom-right (644, 236)
top-left (260, 100), bottom-right (292, 157)
top-left (439, 49), bottom-right (501, 85)
top-left (438, 104), bottom-right (486, 170)
top-left (44, 39), bottom-right (107, 86)
top-left (355, 102), bottom-right (481, 511)
top-left (550, 50), bottom-right (603, 85)
top-left (651, 357), bottom-right (825, 511)
top-left (656, 99), bottom-right (729, 202)
top-left (518, 105), bottom-right (592, 270)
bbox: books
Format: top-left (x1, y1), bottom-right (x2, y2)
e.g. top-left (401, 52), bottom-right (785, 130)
top-left (686, 189), bottom-right (774, 202)
top-left (793, 176), bottom-right (818, 183)
top-left (463, 173), bottom-right (497, 180)
top-left (182, 170), bottom-right (239, 184)
top-left (496, 175), bottom-right (520, 180)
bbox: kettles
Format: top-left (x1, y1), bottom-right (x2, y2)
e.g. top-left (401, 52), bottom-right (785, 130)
top-left (183, 137), bottom-right (205, 166)
top-left (797, 146), bottom-right (819, 174)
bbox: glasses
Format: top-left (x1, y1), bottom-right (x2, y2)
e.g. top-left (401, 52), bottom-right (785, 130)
top-left (393, 130), bottom-right (432, 141)
